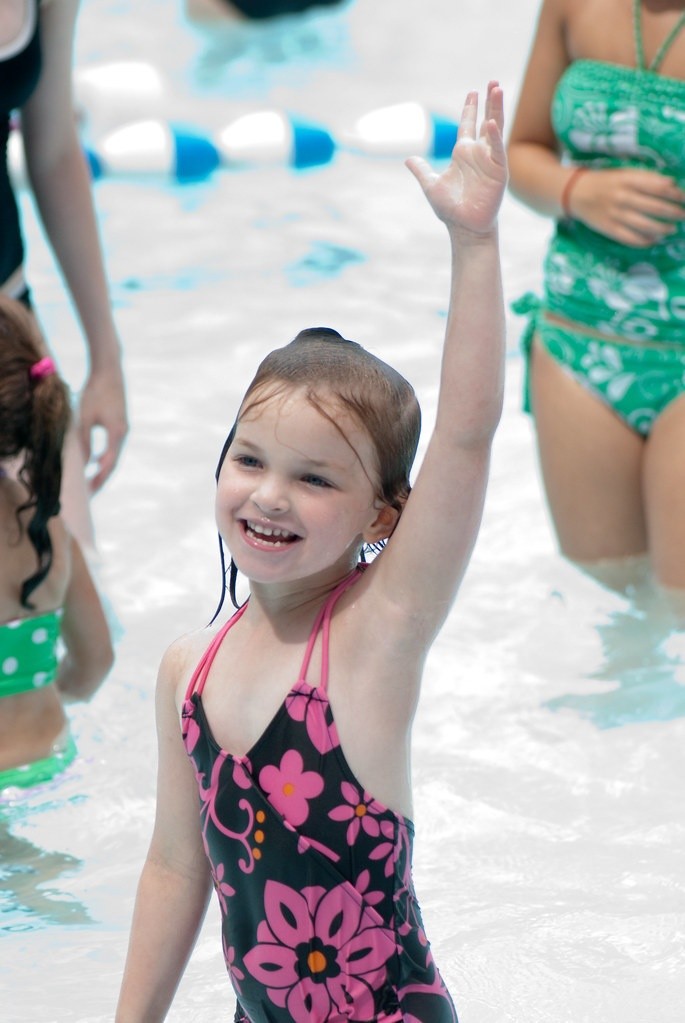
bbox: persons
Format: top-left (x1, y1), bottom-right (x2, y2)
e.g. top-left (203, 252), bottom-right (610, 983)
top-left (0, 300), bottom-right (113, 785)
top-left (505, 0), bottom-right (685, 594)
top-left (0, 0), bottom-right (126, 491)
top-left (117, 81), bottom-right (509, 1023)
